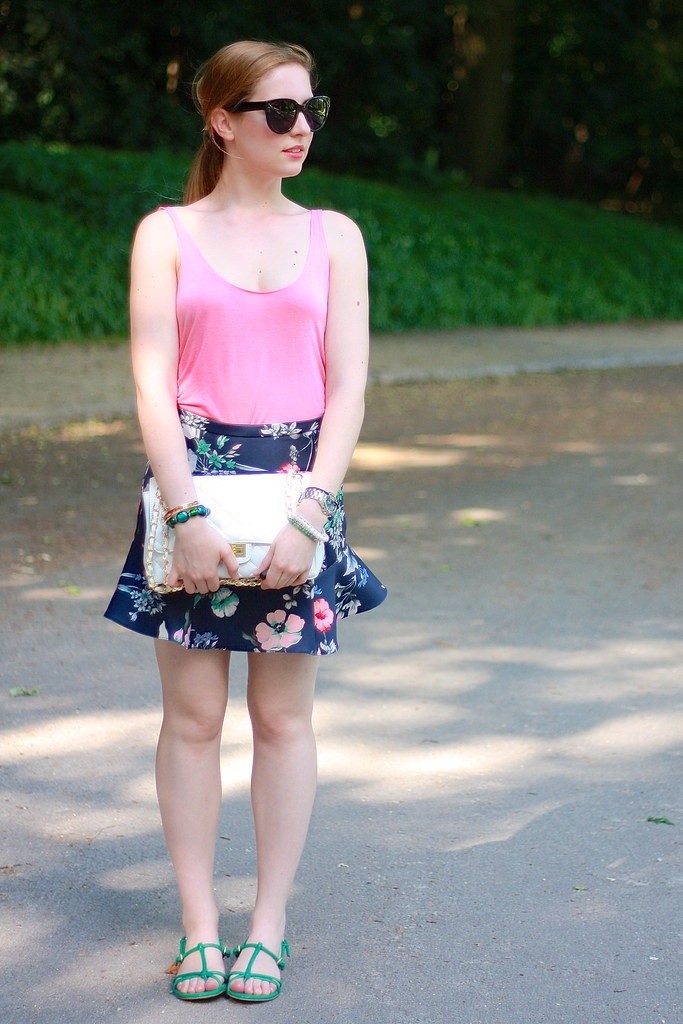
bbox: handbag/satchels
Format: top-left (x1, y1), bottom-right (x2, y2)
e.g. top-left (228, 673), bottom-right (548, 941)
top-left (140, 471), bottom-right (328, 595)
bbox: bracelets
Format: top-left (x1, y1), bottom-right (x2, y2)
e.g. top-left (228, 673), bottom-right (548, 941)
top-left (164, 501), bottom-right (210, 528)
top-left (287, 512), bottom-right (329, 544)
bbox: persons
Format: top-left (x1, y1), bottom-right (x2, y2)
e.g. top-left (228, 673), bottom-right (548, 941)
top-left (103, 40), bottom-right (387, 1003)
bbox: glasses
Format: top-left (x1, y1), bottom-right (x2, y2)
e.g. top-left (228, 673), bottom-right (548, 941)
top-left (233, 95), bottom-right (331, 135)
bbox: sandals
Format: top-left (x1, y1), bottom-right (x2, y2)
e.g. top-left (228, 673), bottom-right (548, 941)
top-left (172, 929), bottom-right (290, 1001)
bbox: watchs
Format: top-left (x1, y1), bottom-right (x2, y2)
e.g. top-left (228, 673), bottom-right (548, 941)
top-left (298, 487), bottom-right (338, 516)
